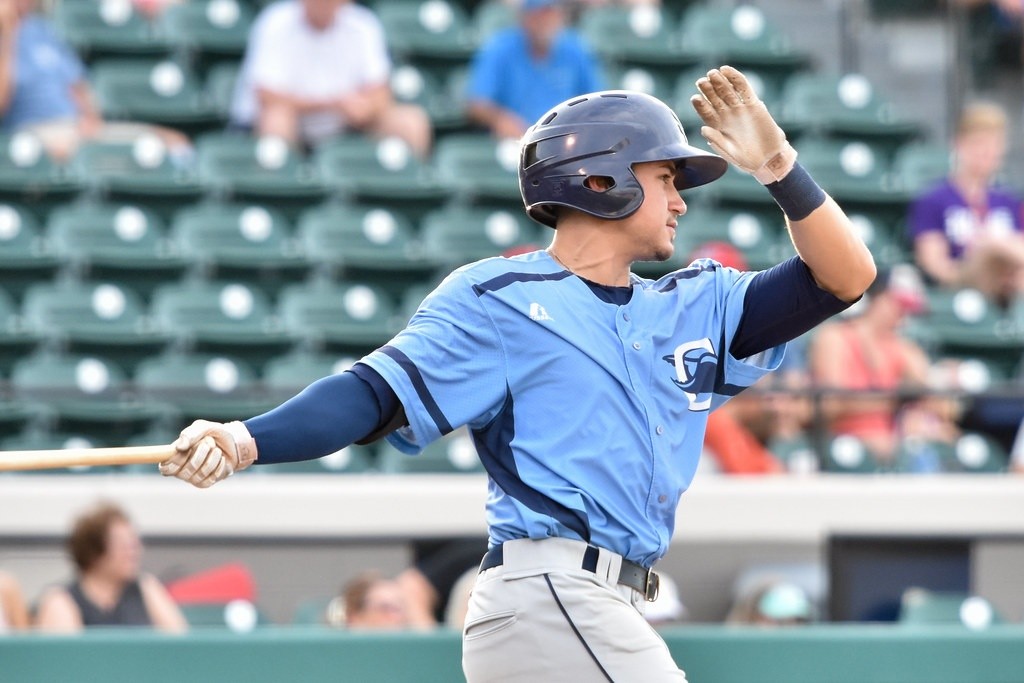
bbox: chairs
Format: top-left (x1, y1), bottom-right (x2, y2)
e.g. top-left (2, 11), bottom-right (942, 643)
top-left (0, 0), bottom-right (1023, 475)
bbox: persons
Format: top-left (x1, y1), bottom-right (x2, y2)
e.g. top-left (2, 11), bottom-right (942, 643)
top-left (0, 0), bottom-right (188, 158)
top-left (337, 540), bottom-right (488, 627)
top-left (226, 0), bottom-right (430, 158)
top-left (160, 66), bottom-right (877, 683)
top-left (32, 503), bottom-right (186, 628)
top-left (686, 100), bottom-right (1024, 476)
top-left (460, 0), bottom-right (607, 173)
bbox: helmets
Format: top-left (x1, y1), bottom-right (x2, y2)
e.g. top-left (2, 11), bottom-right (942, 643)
top-left (517, 90), bottom-right (729, 230)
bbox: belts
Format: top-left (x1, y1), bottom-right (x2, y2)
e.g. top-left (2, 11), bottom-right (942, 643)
top-left (477, 542), bottom-right (660, 602)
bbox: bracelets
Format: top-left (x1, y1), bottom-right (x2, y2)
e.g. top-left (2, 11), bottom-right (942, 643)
top-left (753, 142), bottom-right (799, 187)
top-left (765, 164), bottom-right (825, 221)
top-left (222, 420), bottom-right (258, 471)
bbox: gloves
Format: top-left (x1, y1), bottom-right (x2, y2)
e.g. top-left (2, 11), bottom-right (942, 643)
top-left (691, 65), bottom-right (798, 186)
top-left (159, 419), bottom-right (259, 489)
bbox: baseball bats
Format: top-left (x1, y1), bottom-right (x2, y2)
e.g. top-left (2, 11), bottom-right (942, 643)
top-left (0, 435), bottom-right (216, 473)
